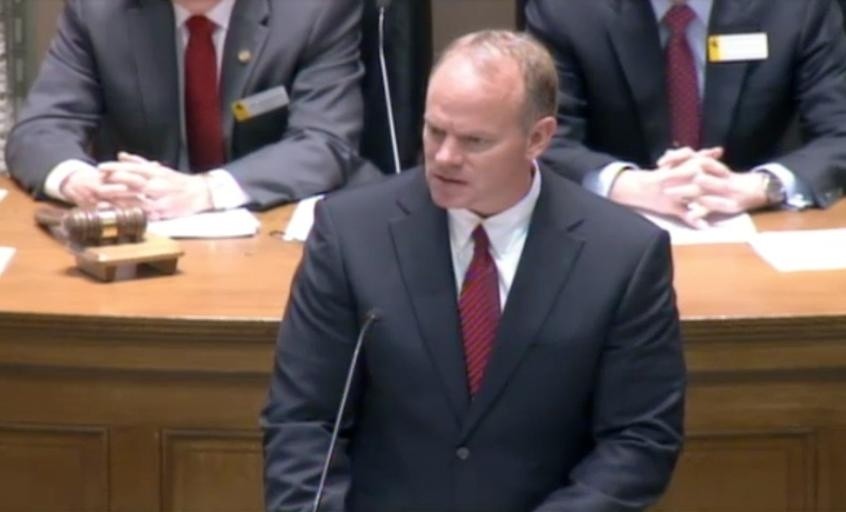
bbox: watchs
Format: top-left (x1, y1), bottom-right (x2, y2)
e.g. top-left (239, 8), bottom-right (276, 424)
top-left (758, 169), bottom-right (785, 209)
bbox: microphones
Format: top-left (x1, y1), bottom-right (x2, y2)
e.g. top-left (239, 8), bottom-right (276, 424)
top-left (376, 1), bottom-right (403, 174)
top-left (312, 308), bottom-right (382, 512)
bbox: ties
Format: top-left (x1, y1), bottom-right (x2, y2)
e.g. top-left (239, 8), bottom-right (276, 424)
top-left (662, 2), bottom-right (704, 155)
top-left (184, 15), bottom-right (226, 175)
top-left (457, 224), bottom-right (505, 404)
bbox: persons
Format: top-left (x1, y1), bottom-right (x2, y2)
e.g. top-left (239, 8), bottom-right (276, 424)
top-left (5, 0), bottom-right (366, 222)
top-left (524, 0), bottom-right (846, 228)
top-left (260, 29), bottom-right (688, 511)
